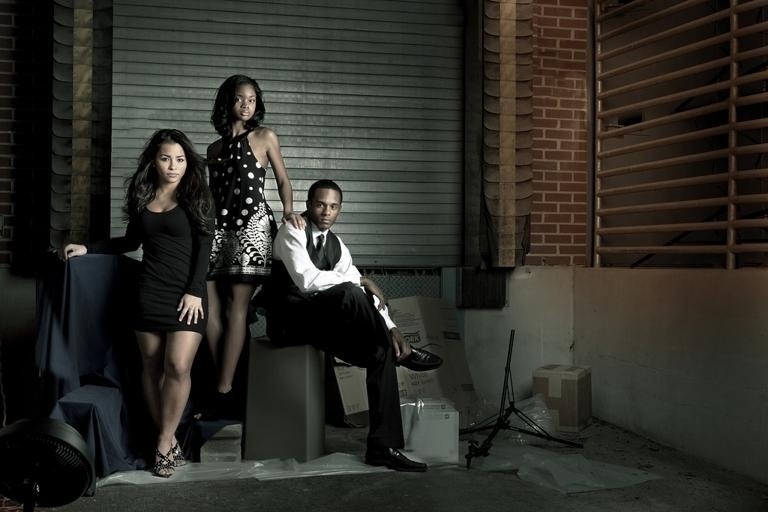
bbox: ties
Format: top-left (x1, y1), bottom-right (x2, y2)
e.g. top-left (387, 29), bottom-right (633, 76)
top-left (317, 234), bottom-right (322, 256)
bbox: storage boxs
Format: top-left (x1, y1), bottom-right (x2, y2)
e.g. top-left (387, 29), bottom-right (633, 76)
top-left (332, 295), bottom-right (477, 425)
top-left (532, 363), bottom-right (592, 434)
top-left (401, 395), bottom-right (460, 465)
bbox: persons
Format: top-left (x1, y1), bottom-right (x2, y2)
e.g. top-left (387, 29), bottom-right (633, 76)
top-left (59, 129), bottom-right (218, 477)
top-left (206, 75), bottom-right (308, 422)
top-left (266, 178), bottom-right (444, 473)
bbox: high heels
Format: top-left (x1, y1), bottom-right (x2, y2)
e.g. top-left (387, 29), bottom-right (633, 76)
top-left (193, 386), bottom-right (234, 422)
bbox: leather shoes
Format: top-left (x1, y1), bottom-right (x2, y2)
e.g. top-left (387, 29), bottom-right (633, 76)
top-left (363, 443), bottom-right (427, 473)
top-left (394, 339), bottom-right (444, 371)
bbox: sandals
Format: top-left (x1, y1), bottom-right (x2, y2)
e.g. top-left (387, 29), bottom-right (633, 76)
top-left (152, 443), bottom-right (175, 479)
top-left (170, 437), bottom-right (186, 467)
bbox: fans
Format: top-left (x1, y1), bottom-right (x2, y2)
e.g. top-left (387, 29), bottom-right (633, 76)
top-left (0, 416), bottom-right (95, 512)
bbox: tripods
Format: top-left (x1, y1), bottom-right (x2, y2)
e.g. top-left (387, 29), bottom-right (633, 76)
top-left (458, 330), bottom-right (584, 468)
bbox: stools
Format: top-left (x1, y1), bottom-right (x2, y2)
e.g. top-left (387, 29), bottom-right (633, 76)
top-left (246, 332), bottom-right (325, 465)
